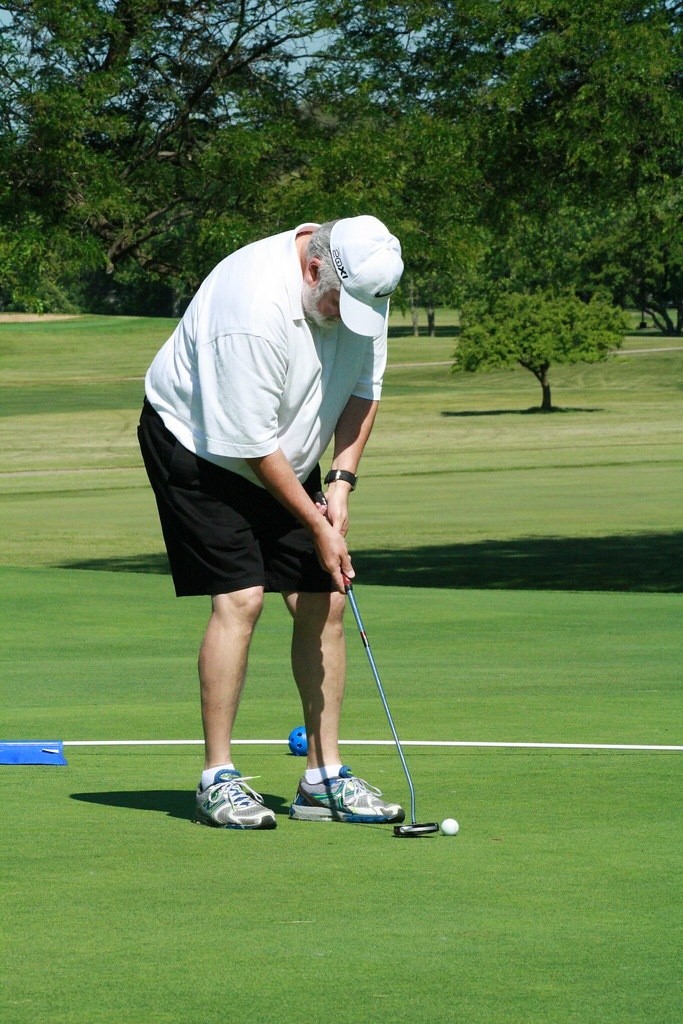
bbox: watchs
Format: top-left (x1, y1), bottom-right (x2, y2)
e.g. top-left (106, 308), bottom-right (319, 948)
top-left (324, 469), bottom-right (358, 492)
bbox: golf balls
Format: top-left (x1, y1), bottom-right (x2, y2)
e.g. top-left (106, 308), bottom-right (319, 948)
top-left (441, 819), bottom-right (460, 836)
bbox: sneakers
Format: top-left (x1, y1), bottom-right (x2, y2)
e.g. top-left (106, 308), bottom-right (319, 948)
top-left (196, 769), bottom-right (278, 829)
top-left (289, 774), bottom-right (407, 823)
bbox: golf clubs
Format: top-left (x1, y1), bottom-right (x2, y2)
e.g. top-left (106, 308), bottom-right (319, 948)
top-left (314, 489), bottom-right (439, 837)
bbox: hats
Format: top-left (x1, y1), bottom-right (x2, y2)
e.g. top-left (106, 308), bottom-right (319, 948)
top-left (331, 215), bottom-right (405, 336)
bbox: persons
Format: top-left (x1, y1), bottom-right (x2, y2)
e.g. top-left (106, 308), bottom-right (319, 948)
top-left (135, 214), bottom-right (406, 831)
